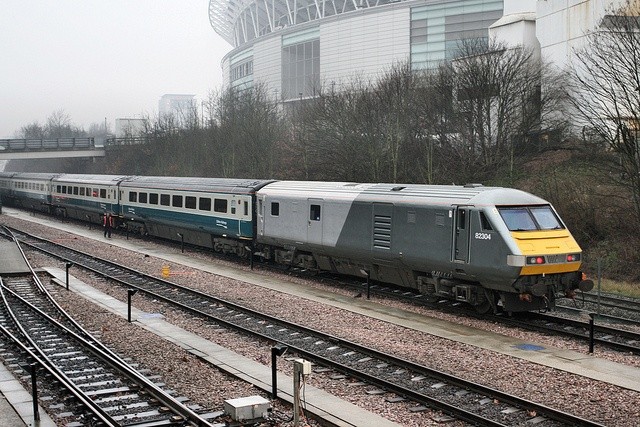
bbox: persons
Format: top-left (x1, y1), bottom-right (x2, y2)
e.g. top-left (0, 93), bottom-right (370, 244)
top-left (101, 211), bottom-right (113, 239)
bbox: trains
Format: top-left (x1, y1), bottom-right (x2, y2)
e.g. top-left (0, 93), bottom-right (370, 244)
top-left (0, 170), bottom-right (595, 316)
